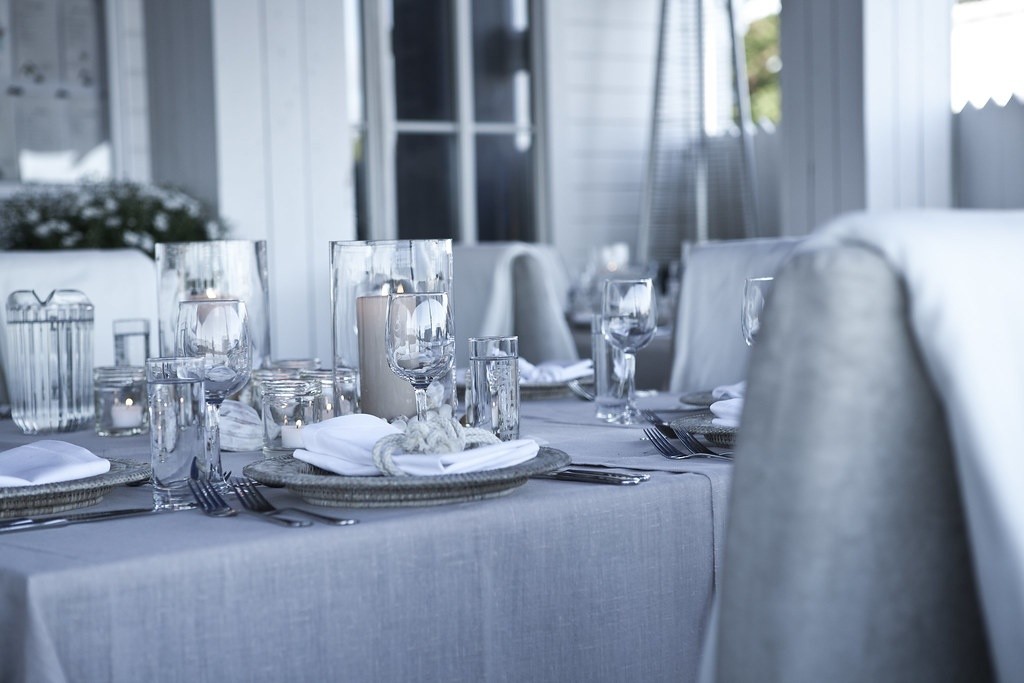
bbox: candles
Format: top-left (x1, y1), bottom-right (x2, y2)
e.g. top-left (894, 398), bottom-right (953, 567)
top-left (186, 289), bottom-right (234, 355)
top-left (354, 284), bottom-right (417, 419)
top-left (110, 398), bottom-right (144, 428)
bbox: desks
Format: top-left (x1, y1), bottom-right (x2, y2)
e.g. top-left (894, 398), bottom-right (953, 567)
top-left (573, 327), bottom-right (670, 392)
top-left (0, 389), bottom-right (735, 683)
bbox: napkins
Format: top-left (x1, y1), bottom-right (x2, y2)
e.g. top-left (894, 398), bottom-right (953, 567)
top-left (490, 347), bottom-right (595, 387)
top-left (711, 381), bottom-right (745, 400)
top-left (293, 412), bottom-right (542, 476)
top-left (0, 439), bottom-right (110, 488)
top-left (708, 396), bottom-right (743, 428)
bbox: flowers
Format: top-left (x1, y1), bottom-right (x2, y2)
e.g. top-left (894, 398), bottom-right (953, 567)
top-left (0, 173), bottom-right (232, 270)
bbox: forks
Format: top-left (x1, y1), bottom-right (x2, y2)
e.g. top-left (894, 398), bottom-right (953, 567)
top-left (186, 474), bottom-right (359, 528)
top-left (640, 409), bottom-right (739, 464)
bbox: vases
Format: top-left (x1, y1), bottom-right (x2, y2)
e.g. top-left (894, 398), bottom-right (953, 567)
top-left (0, 247), bottom-right (201, 400)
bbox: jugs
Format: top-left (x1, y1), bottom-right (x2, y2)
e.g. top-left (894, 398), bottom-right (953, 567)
top-left (4, 289), bottom-right (94, 434)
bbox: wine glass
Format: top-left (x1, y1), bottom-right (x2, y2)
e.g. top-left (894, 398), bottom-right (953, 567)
top-left (600, 279), bottom-right (658, 426)
top-left (174, 299), bottom-right (254, 492)
top-left (383, 291), bottom-right (455, 428)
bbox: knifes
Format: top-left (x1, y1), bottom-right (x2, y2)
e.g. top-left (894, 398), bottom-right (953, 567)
top-left (0, 501), bottom-right (199, 536)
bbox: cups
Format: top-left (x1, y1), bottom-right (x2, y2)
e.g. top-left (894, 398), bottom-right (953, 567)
top-left (467, 335), bottom-right (519, 443)
top-left (740, 278), bottom-right (780, 346)
top-left (145, 355), bottom-right (209, 506)
top-left (589, 315), bottom-right (636, 418)
top-left (93, 315), bottom-right (358, 442)
top-left (152, 237), bottom-right (271, 382)
top-left (329, 238), bottom-right (455, 429)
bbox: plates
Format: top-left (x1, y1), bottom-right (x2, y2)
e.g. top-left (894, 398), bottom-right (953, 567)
top-left (0, 457), bottom-right (153, 519)
top-left (668, 412), bottom-right (744, 449)
top-left (243, 443), bottom-right (572, 509)
top-left (450, 367), bottom-right (594, 400)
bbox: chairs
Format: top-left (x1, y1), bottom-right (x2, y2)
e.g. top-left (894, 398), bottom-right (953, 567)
top-left (717, 207), bottom-right (1024, 683)
top-left (667, 237), bottom-right (814, 395)
top-left (449, 240), bottom-right (579, 364)
